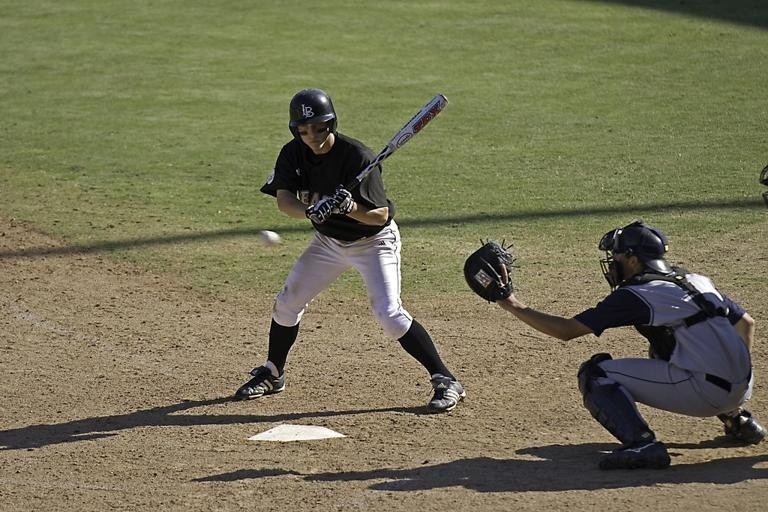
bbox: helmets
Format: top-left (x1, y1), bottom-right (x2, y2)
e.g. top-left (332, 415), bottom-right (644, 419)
top-left (288, 86), bottom-right (338, 127)
top-left (612, 220), bottom-right (675, 277)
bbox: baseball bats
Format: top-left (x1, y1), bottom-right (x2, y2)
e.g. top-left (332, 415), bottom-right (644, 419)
top-left (312, 94), bottom-right (449, 222)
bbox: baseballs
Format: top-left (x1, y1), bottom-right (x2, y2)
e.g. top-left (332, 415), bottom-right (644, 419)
top-left (258, 232), bottom-right (279, 245)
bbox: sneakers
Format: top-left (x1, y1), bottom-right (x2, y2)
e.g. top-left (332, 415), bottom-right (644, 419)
top-left (722, 409), bottom-right (767, 445)
top-left (234, 366), bottom-right (286, 400)
top-left (598, 440), bottom-right (671, 472)
top-left (426, 373), bottom-right (468, 414)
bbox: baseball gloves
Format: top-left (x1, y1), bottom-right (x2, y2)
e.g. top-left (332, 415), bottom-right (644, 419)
top-left (466, 240), bottom-right (517, 302)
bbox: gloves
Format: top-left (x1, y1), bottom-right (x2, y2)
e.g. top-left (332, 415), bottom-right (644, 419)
top-left (332, 185), bottom-right (356, 215)
top-left (304, 198), bottom-right (333, 224)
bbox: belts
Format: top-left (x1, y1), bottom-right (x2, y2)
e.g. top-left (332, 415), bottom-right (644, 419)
top-left (701, 367), bottom-right (755, 393)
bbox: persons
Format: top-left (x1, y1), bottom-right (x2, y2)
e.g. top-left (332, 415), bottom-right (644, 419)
top-left (232, 86), bottom-right (467, 414)
top-left (463, 220), bottom-right (767, 472)
